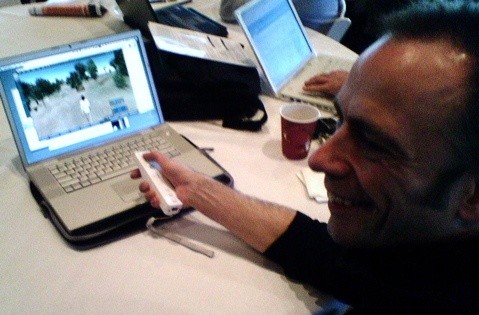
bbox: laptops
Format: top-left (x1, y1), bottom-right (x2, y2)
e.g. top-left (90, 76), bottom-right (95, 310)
top-left (0, 30), bottom-right (225, 236)
top-left (114, 0), bottom-right (227, 41)
top-left (233, 0), bottom-right (356, 116)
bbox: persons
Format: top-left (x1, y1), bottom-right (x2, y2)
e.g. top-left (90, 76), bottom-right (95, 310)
top-left (128, 0), bottom-right (479, 314)
top-left (79, 95), bottom-right (94, 126)
top-left (302, 68), bottom-right (349, 96)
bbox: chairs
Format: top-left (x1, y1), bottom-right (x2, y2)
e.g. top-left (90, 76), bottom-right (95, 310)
top-left (288, 0), bottom-right (353, 42)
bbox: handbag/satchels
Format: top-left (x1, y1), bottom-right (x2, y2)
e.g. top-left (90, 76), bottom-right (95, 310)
top-left (144, 48), bottom-right (263, 123)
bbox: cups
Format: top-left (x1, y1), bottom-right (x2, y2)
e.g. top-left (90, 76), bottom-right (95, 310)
top-left (280, 103), bottom-right (321, 159)
